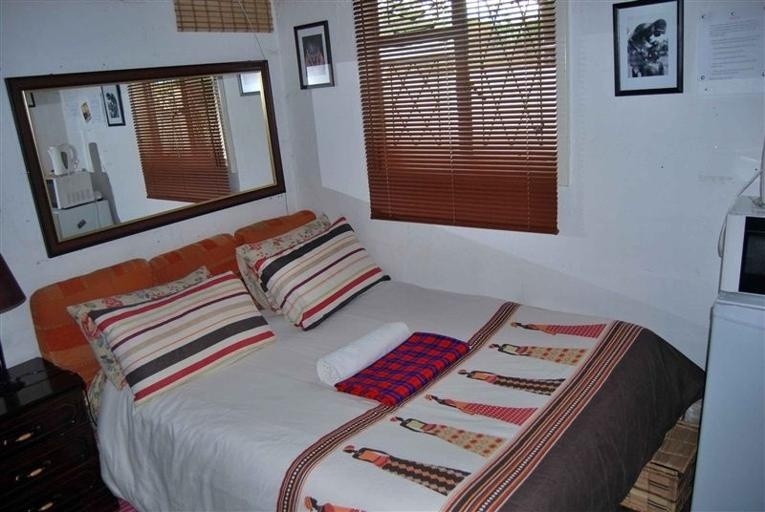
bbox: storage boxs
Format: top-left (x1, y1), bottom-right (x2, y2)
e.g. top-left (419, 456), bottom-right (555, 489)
top-left (620, 419), bottom-right (700, 512)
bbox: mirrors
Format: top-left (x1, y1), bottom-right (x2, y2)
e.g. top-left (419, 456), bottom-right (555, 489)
top-left (4, 59), bottom-right (286, 257)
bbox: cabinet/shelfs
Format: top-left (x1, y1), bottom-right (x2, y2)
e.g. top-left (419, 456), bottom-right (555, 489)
top-left (52, 199), bottom-right (114, 241)
top-left (688, 293), bottom-right (765, 512)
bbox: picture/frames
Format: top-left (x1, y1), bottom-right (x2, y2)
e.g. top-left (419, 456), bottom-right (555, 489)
top-left (294, 20), bottom-right (334, 90)
top-left (236, 72), bottom-right (260, 96)
top-left (25, 91), bottom-right (36, 107)
top-left (612, 0), bottom-right (684, 97)
top-left (101, 84), bottom-right (126, 127)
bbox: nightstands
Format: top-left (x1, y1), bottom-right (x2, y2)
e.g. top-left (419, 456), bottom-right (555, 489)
top-left (0, 358), bottom-right (120, 512)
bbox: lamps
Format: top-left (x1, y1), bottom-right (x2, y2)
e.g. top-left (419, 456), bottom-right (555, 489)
top-left (0, 253), bottom-right (26, 394)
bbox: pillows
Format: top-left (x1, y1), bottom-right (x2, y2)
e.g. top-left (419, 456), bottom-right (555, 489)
top-left (255, 216), bottom-right (390, 330)
top-left (89, 268), bottom-right (276, 407)
top-left (235, 214), bottom-right (332, 314)
top-left (67, 263), bottom-right (213, 392)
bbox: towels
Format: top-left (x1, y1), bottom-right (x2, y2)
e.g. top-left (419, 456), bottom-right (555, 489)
top-left (336, 332), bottom-right (469, 406)
top-left (316, 320), bottom-right (411, 385)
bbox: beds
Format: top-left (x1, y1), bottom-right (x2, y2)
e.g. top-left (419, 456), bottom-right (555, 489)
top-left (29, 208), bottom-right (706, 512)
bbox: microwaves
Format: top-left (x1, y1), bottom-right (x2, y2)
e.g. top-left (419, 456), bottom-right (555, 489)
top-left (40, 166), bottom-right (96, 210)
top-left (716, 192), bottom-right (764, 296)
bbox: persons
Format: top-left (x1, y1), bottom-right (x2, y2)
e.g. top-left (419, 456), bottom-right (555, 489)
top-left (303, 495), bottom-right (367, 512)
top-left (640, 44), bottom-right (665, 76)
top-left (391, 416), bottom-right (508, 459)
top-left (342, 445), bottom-right (471, 497)
top-left (626, 19), bottom-right (668, 77)
top-left (458, 367), bottom-right (568, 397)
top-left (490, 341), bottom-right (589, 367)
top-left (425, 393), bottom-right (540, 428)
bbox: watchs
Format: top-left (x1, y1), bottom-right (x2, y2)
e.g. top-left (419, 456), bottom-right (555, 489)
top-left (511, 319), bottom-right (608, 339)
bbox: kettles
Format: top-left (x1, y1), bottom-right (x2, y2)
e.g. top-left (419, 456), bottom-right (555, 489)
top-left (44, 144), bottom-right (78, 179)
